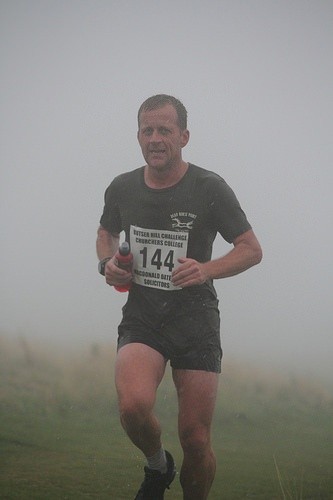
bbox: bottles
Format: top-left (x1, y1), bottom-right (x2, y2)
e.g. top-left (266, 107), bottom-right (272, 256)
top-left (114, 242), bottom-right (133, 292)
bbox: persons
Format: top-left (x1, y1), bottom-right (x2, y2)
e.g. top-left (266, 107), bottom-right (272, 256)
top-left (96, 94), bottom-right (263, 500)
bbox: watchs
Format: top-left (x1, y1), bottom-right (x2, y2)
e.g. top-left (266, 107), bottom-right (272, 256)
top-left (98, 257), bottom-right (112, 276)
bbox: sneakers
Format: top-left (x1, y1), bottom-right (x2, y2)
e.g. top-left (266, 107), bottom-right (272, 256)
top-left (135, 451), bottom-right (176, 500)
